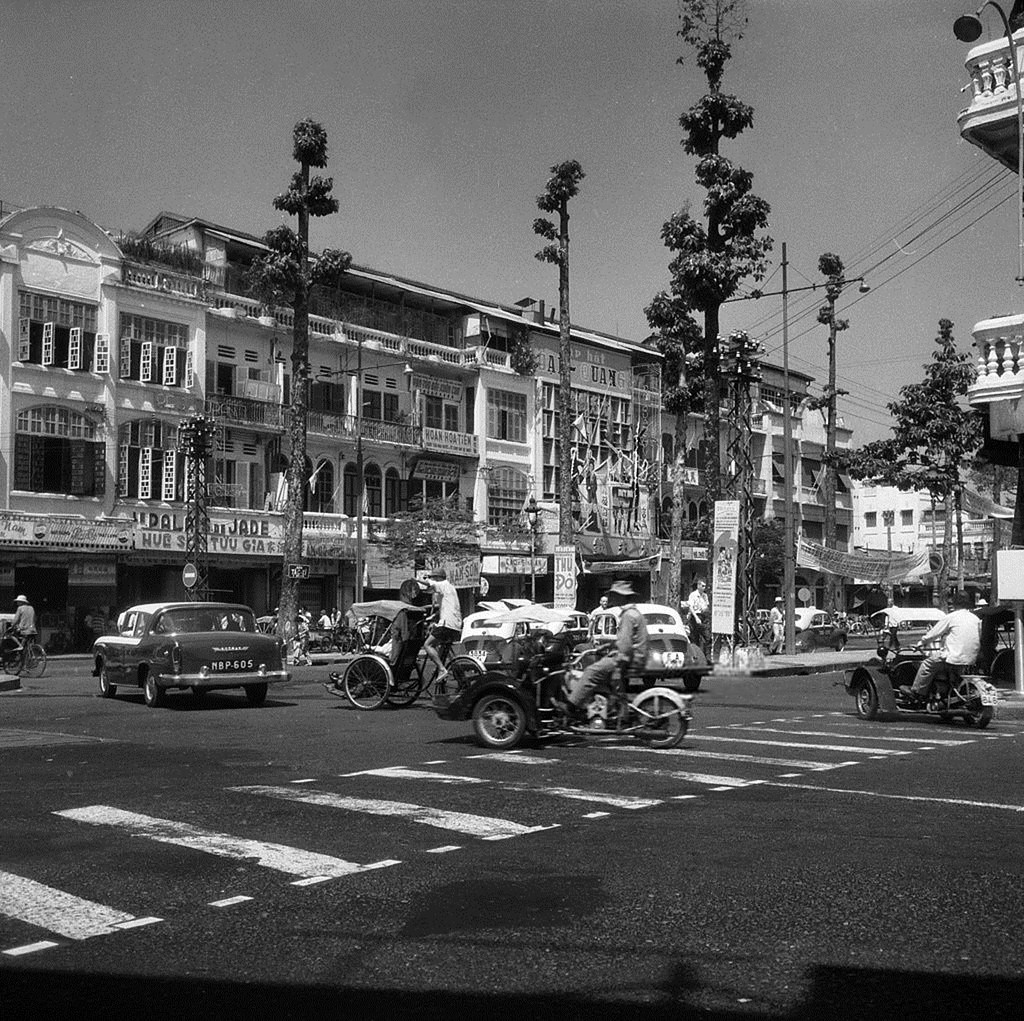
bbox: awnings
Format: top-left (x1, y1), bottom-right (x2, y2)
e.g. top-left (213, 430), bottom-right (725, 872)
top-left (838, 473), bottom-right (855, 489)
top-left (773, 455), bottom-right (784, 478)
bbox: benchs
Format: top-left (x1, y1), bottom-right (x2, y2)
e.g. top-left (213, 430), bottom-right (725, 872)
top-left (889, 646), bottom-right (941, 684)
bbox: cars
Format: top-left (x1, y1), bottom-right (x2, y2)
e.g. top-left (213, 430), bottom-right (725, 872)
top-left (587, 602), bottom-right (715, 691)
top-left (794, 605), bottom-right (849, 654)
top-left (90, 601), bottom-right (293, 709)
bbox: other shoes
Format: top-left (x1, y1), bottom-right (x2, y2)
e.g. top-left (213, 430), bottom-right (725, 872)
top-left (12, 647), bottom-right (23, 651)
top-left (435, 668), bottom-right (447, 684)
top-left (769, 648), bottom-right (773, 654)
top-left (551, 698), bottom-right (574, 717)
top-left (900, 685), bottom-right (917, 700)
top-left (777, 652), bottom-right (784, 655)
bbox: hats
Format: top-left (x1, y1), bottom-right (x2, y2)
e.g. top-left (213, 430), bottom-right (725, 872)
top-left (605, 580), bottom-right (637, 596)
top-left (14, 594), bottom-right (28, 602)
top-left (428, 567), bottom-right (446, 576)
top-left (773, 597), bottom-right (785, 602)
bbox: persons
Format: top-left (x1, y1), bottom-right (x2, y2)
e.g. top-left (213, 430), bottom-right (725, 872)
top-left (559, 580), bottom-right (650, 718)
top-left (769, 597), bottom-right (786, 653)
top-left (293, 607), bottom-right (313, 666)
top-left (329, 638), bottom-right (392, 685)
top-left (8, 595), bottom-right (37, 651)
top-left (718, 547), bottom-right (732, 575)
top-left (586, 597), bottom-right (608, 638)
top-left (345, 610), bottom-right (371, 645)
top-left (686, 579), bottom-right (712, 658)
top-left (883, 598), bottom-right (901, 648)
top-left (899, 588), bottom-right (982, 705)
top-left (318, 607), bottom-right (342, 626)
top-left (412, 569), bottom-right (462, 684)
top-left (84, 609), bottom-right (105, 638)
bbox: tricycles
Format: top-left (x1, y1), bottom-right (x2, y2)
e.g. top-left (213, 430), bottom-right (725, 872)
top-left (430, 618), bottom-right (696, 748)
top-left (843, 608), bottom-right (997, 728)
top-left (322, 600), bottom-right (489, 712)
top-left (253, 605), bottom-right (355, 657)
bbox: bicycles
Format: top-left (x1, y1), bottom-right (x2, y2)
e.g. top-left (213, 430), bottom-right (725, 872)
top-left (0, 622), bottom-right (48, 680)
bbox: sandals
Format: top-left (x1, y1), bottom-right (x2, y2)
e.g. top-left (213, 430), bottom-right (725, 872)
top-left (330, 672), bottom-right (341, 689)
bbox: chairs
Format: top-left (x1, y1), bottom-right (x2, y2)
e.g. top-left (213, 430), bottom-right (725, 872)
top-left (373, 618), bottom-right (426, 679)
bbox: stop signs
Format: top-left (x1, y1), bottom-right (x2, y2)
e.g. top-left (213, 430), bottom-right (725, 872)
top-left (182, 563), bottom-right (198, 588)
